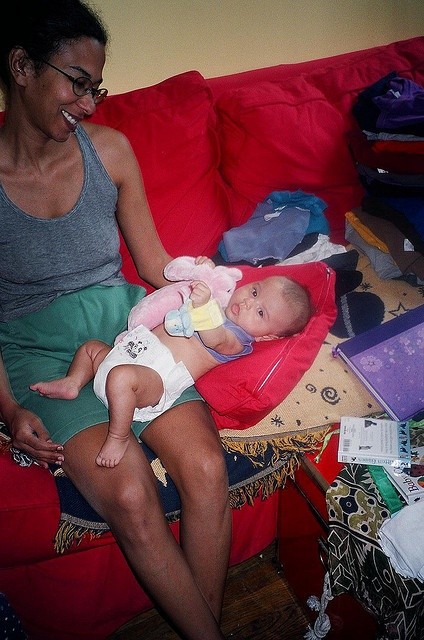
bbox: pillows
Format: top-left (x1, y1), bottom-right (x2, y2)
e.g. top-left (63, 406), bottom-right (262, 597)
top-left (218, 243), bottom-right (424, 468)
top-left (196, 261), bottom-right (338, 428)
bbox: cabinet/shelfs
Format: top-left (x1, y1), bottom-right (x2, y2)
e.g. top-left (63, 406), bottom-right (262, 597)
top-left (269, 426), bottom-right (377, 640)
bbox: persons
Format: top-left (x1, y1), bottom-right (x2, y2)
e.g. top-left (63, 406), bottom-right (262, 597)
top-left (25, 253), bottom-right (314, 469)
top-left (1, 0), bottom-right (234, 640)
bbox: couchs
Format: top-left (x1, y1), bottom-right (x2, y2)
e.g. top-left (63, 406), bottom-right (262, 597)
top-left (0, 37), bottom-right (424, 640)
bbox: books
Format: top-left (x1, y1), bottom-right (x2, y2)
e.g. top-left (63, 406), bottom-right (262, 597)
top-left (382, 446), bottom-right (423, 506)
top-left (332, 303), bottom-right (424, 422)
top-left (336, 414), bottom-right (412, 473)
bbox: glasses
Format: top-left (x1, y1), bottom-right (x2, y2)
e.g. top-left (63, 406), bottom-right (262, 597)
top-left (26, 55), bottom-right (108, 104)
